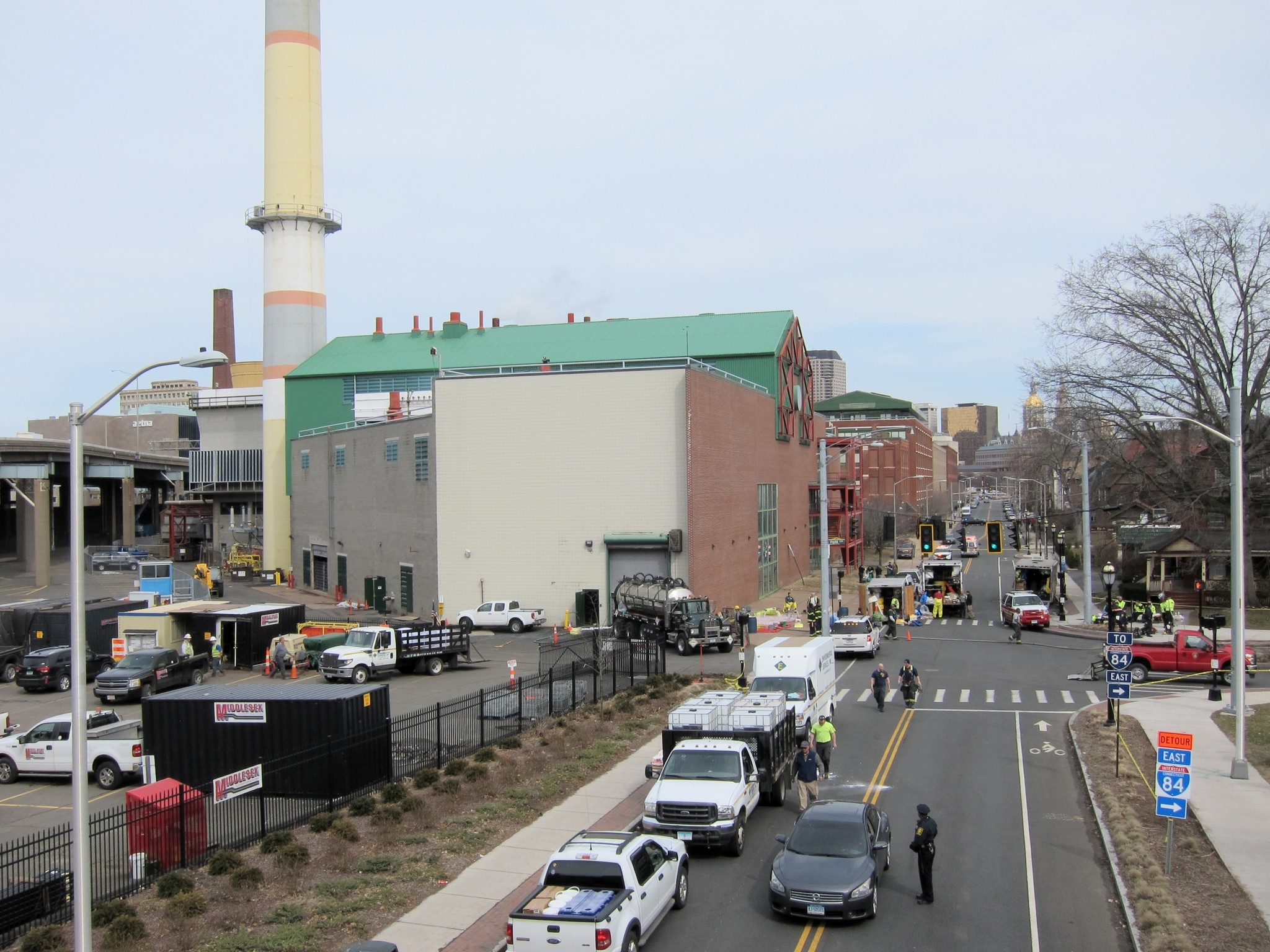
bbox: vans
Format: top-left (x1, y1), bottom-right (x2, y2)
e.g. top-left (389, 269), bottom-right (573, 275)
top-left (1002, 590), bottom-right (1050, 629)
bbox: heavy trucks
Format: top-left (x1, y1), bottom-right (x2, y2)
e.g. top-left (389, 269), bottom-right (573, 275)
top-left (610, 573), bottom-right (736, 656)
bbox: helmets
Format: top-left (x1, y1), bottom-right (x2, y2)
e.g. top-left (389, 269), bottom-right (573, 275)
top-left (735, 605), bottom-right (740, 610)
top-left (209, 636), bottom-right (217, 641)
top-left (184, 633), bottom-right (192, 639)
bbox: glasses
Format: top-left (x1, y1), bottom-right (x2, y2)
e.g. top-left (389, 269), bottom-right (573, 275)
top-left (819, 717), bottom-right (826, 720)
top-left (801, 747), bottom-right (807, 749)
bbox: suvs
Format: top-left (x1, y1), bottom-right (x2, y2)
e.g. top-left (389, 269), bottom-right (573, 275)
top-left (828, 613), bottom-right (882, 658)
top-left (14, 644), bottom-right (118, 695)
top-left (960, 505), bottom-right (987, 527)
top-left (90, 550), bottom-right (141, 571)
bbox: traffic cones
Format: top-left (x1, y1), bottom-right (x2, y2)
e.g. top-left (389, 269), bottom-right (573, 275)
top-left (287, 659), bottom-right (298, 680)
top-left (906, 631), bottom-right (911, 640)
top-left (567, 622), bottom-right (572, 631)
top-left (347, 598), bottom-right (369, 615)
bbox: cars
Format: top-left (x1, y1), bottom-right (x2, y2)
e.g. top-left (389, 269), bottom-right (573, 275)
top-left (769, 801), bottom-right (893, 926)
top-left (970, 494), bottom-right (990, 509)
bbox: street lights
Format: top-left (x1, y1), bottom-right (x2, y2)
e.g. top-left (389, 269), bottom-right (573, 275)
top-left (1027, 426), bottom-right (1093, 624)
top-left (1102, 561), bottom-right (1115, 632)
top-left (1003, 475), bottom-right (1050, 558)
top-left (892, 474), bottom-right (982, 562)
top-left (986, 475), bottom-right (998, 498)
top-left (66, 348), bottom-right (230, 951)
top-left (1137, 387), bottom-right (1251, 780)
top-left (821, 434), bottom-right (886, 636)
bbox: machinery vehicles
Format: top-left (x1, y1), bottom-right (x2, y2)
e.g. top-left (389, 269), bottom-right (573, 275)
top-left (193, 563), bottom-right (224, 598)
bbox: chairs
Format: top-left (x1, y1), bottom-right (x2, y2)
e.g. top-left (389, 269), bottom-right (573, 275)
top-left (1171, 611), bottom-right (1185, 631)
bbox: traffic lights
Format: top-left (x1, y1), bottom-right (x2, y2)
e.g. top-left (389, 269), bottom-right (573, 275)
top-left (1193, 579), bottom-right (1205, 591)
top-left (986, 520), bottom-right (1003, 552)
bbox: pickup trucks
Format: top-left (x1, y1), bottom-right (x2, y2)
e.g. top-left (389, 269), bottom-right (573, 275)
top-left (456, 600), bottom-right (547, 633)
top-left (1103, 629), bottom-right (1257, 687)
top-left (504, 829), bottom-right (692, 952)
top-left (109, 546), bottom-right (149, 562)
top-left (0, 647), bottom-right (210, 790)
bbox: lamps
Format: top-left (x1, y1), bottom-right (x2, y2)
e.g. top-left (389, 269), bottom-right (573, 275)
top-left (466, 549), bottom-right (471, 558)
top-left (585, 540), bottom-right (593, 546)
top-left (795, 526), bottom-right (797, 529)
top-left (337, 541), bottom-right (343, 546)
top-left (289, 535), bottom-right (293, 539)
top-left (732, 540), bottom-right (735, 543)
top-left (712, 544), bottom-right (716, 548)
top-left (748, 536), bottom-right (751, 540)
top-left (783, 529), bottom-right (786, 532)
top-left (805, 524), bottom-right (807, 527)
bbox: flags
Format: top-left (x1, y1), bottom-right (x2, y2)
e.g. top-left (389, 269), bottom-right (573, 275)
top-left (431, 602), bottom-right (441, 627)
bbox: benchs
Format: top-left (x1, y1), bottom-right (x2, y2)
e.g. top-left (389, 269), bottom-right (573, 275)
top-left (1137, 613), bottom-right (1161, 622)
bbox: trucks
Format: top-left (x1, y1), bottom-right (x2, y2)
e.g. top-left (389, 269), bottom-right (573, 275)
top-left (1013, 554), bottom-right (1059, 605)
top-left (640, 635), bottom-right (837, 859)
top-left (865, 559), bottom-right (966, 628)
top-left (318, 623), bottom-right (473, 685)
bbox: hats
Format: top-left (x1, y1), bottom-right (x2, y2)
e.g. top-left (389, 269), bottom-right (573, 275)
top-left (800, 741), bottom-right (809, 748)
top-left (917, 804), bottom-right (930, 816)
top-left (966, 591), bottom-right (970, 593)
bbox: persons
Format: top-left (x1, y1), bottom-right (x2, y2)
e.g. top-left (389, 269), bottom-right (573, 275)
top-left (209, 636), bottom-right (224, 677)
top-left (909, 804), bottom-right (937, 905)
top-left (182, 634), bottom-right (193, 659)
top-left (269, 637), bottom-right (295, 680)
top-left (1009, 608), bottom-right (1023, 645)
top-left (1102, 595), bottom-right (1174, 638)
top-left (733, 560), bottom-right (974, 814)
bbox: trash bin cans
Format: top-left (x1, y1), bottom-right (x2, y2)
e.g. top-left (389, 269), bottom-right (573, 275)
top-left (949, 522), bottom-right (953, 529)
top-left (838, 607), bottom-right (849, 618)
top-left (747, 617), bottom-right (757, 633)
top-left (260, 570), bottom-right (276, 582)
top-left (231, 566), bottom-right (253, 581)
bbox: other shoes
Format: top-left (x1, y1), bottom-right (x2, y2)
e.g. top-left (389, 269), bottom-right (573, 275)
top-left (1147, 634), bottom-right (1153, 637)
top-left (825, 772), bottom-right (828, 779)
top-left (905, 704), bottom-right (911, 708)
top-left (877, 704), bottom-right (881, 708)
top-left (910, 704), bottom-right (914, 709)
top-left (1008, 637), bottom-right (1013, 643)
top-left (1016, 642), bottom-right (1022, 644)
top-left (918, 899), bottom-right (934, 904)
top-left (1141, 628), bottom-right (1145, 634)
top-left (884, 636), bottom-right (889, 640)
top-left (816, 632), bottom-right (822, 635)
top-left (810, 634), bottom-right (817, 637)
top-left (916, 893), bottom-right (925, 898)
top-left (893, 637), bottom-right (899, 640)
top-left (880, 707), bottom-right (884, 711)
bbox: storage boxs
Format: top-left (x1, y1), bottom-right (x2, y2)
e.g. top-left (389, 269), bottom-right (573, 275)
top-left (522, 898), bottom-right (551, 914)
top-left (536, 885), bottom-right (566, 900)
top-left (943, 595), bottom-right (959, 603)
top-left (873, 597), bottom-right (884, 613)
